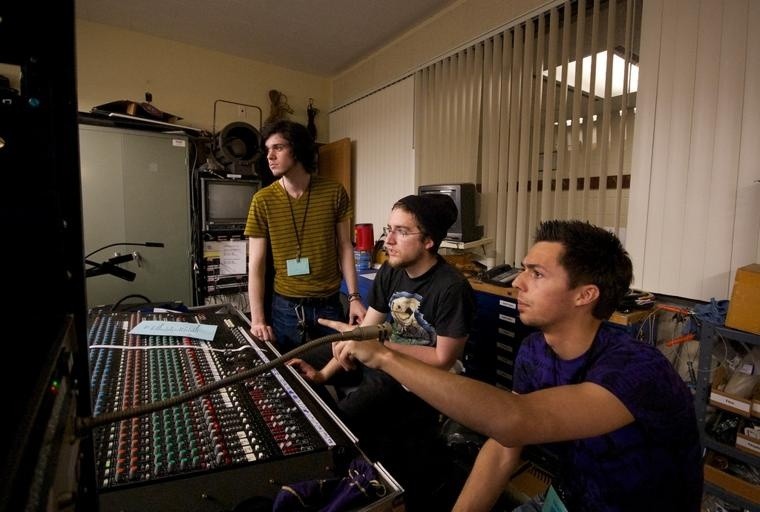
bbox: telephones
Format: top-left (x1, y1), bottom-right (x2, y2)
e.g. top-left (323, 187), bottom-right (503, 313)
top-left (481, 264), bottom-right (525, 287)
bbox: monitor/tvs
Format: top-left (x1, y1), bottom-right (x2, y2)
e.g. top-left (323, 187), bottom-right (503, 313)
top-left (419, 183), bottom-right (484, 242)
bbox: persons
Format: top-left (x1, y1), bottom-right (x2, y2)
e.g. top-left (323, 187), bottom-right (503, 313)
top-left (311, 218), bottom-right (704, 512)
top-left (286, 193), bottom-right (476, 470)
top-left (241, 121), bottom-right (366, 344)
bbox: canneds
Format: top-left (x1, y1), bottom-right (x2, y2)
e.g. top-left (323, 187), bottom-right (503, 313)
top-left (355, 223), bottom-right (375, 251)
top-left (352, 251), bottom-right (372, 271)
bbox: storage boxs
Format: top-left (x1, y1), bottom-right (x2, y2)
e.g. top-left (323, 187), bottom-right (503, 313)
top-left (725, 264), bottom-right (760, 335)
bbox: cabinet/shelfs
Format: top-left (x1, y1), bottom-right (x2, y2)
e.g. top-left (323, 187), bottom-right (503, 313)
top-left (695, 321), bottom-right (760, 512)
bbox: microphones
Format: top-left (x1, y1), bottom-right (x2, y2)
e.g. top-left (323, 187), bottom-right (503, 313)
top-left (89, 321), bottom-right (392, 426)
top-left (84, 242), bottom-right (164, 260)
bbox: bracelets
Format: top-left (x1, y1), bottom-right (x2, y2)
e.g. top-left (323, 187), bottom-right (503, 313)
top-left (347, 291), bottom-right (362, 303)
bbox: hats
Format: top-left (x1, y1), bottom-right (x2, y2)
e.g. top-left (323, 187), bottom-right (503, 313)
top-left (399, 193), bottom-right (461, 251)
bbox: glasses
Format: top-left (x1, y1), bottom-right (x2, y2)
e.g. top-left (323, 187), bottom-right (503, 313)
top-left (383, 226), bottom-right (423, 238)
